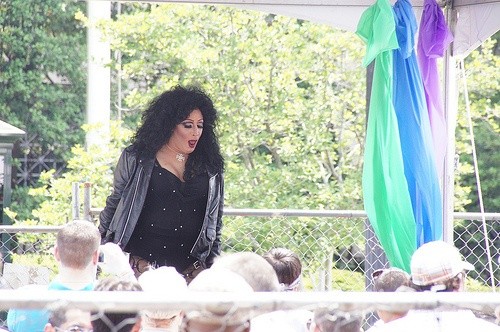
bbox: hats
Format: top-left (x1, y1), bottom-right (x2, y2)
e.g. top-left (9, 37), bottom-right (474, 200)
top-left (409, 241), bottom-right (476, 286)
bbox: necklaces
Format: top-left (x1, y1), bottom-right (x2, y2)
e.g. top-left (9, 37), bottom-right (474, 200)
top-left (167, 144), bottom-right (190, 164)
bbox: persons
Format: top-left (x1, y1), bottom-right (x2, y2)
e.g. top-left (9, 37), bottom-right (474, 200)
top-left (44, 247), bottom-right (365, 332)
top-left (98, 84), bottom-right (225, 286)
top-left (367, 240), bottom-right (500, 332)
top-left (7, 219), bottom-right (101, 332)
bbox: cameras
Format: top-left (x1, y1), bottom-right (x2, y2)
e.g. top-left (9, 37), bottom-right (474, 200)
top-left (97, 245), bottom-right (105, 263)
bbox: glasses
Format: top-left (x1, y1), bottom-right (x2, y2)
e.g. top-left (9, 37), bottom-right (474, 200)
top-left (371, 269), bottom-right (385, 288)
